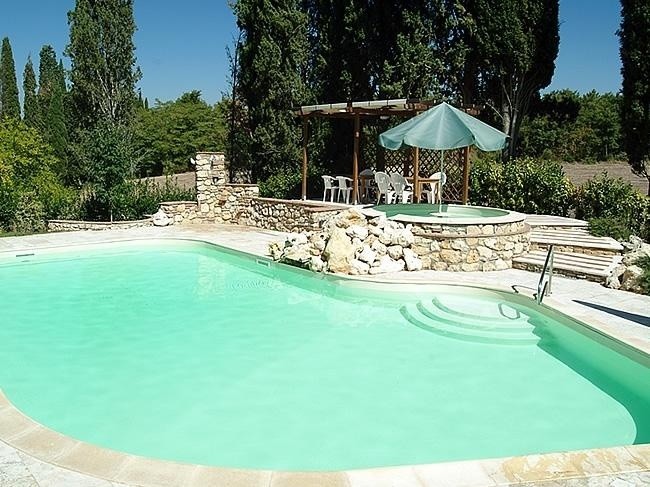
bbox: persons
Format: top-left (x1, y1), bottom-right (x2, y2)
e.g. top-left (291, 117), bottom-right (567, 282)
top-left (419, 168), bottom-right (432, 202)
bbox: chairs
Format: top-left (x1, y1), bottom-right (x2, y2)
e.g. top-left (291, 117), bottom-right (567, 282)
top-left (320, 168), bottom-right (447, 205)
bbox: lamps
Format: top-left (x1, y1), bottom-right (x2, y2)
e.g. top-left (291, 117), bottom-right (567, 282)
top-left (353, 128), bottom-right (361, 139)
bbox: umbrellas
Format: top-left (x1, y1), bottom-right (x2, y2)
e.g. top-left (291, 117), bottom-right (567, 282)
top-left (377, 102), bottom-right (510, 217)
